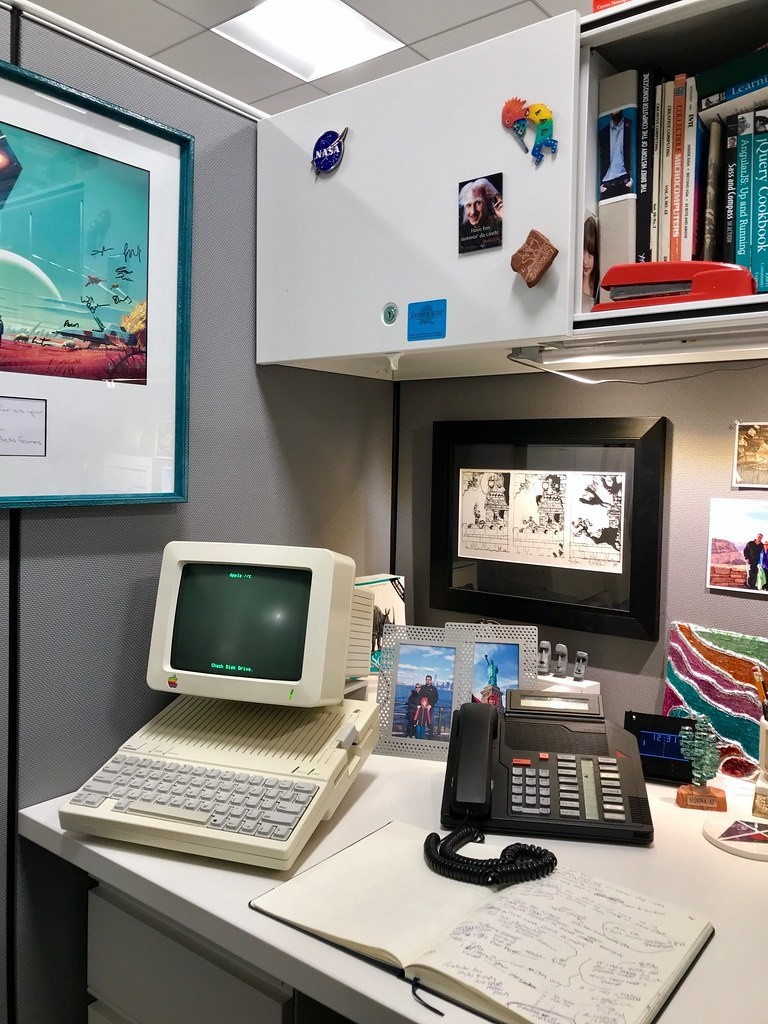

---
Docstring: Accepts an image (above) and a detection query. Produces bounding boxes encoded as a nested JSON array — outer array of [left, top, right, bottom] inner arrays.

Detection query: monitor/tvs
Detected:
[[146, 539, 375, 709]]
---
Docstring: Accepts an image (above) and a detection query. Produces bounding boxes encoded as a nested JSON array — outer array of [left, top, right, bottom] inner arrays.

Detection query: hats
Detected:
[[421, 697, 428, 703], [763, 541, 768, 544], [487, 695, 498, 706]]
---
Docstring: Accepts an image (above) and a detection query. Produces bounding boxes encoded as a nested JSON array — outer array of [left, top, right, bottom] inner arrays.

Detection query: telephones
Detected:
[[439, 700, 656, 848]]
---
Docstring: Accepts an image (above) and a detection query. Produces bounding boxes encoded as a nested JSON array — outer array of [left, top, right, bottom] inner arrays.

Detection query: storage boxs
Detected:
[[354, 572, 406, 672]]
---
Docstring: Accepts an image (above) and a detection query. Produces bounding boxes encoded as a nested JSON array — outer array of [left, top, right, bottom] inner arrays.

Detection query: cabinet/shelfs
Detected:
[[254, 0, 768, 383]]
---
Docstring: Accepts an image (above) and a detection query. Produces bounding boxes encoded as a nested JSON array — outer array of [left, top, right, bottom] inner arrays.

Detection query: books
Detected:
[[598, 45, 768, 304], [248, 821, 715, 1024]]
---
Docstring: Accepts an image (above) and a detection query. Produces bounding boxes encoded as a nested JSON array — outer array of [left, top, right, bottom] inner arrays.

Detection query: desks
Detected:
[[17, 753, 768, 1024]]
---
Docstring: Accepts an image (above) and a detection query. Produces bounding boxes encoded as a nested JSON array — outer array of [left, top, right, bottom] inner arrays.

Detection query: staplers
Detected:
[[589, 261, 757, 314]]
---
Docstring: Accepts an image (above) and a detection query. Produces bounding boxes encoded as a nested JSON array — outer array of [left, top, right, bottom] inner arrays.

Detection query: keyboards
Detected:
[[59, 730, 350, 870]]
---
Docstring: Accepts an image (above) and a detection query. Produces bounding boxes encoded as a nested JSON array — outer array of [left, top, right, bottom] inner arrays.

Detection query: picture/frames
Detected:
[[445, 622, 538, 712], [374, 623, 476, 759], [0, 59, 196, 508], [429, 415, 666, 643]]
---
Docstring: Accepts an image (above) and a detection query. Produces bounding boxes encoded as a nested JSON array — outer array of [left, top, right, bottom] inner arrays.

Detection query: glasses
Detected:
[[416, 687, 420, 688]]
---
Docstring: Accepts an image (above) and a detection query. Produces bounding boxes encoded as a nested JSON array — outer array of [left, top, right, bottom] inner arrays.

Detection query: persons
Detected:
[[459, 178, 503, 253], [582, 216, 598, 298], [743, 534, 768, 591], [485, 655, 498, 687], [406, 675, 438, 739], [598, 110, 633, 201]]
